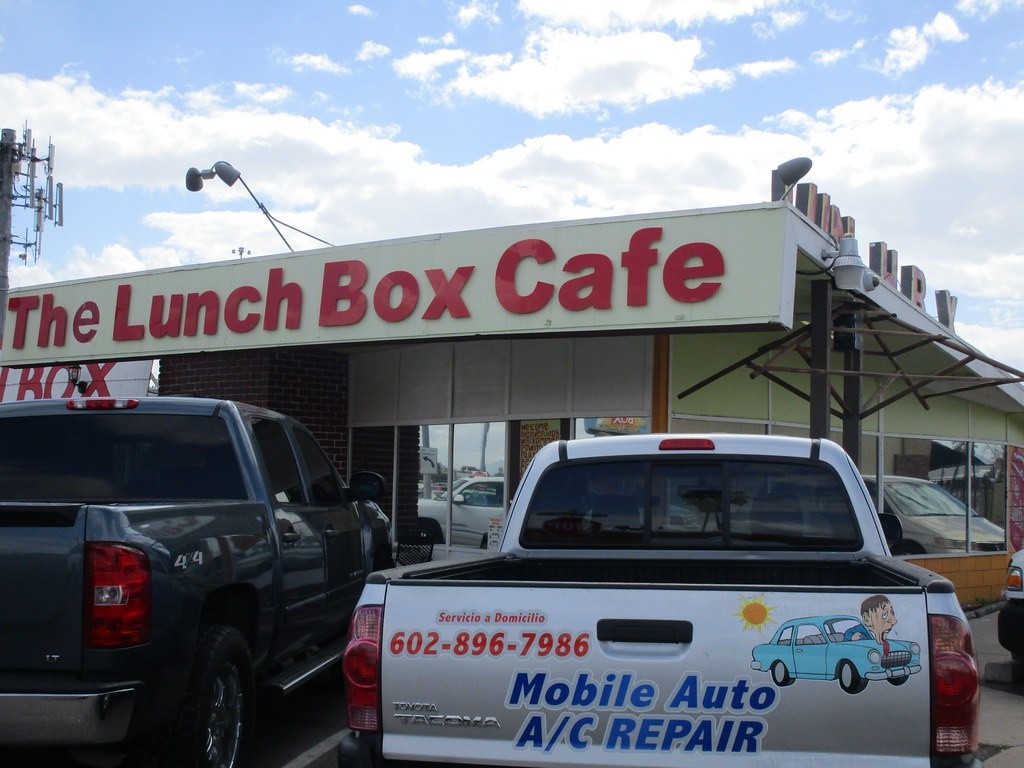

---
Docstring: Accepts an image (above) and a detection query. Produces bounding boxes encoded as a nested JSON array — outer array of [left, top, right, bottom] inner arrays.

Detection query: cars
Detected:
[[997, 548, 1024, 660], [782, 469, 1008, 558], [418, 471, 507, 552]]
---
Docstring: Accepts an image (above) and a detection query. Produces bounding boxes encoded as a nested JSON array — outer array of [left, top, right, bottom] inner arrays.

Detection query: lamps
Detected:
[[822, 233, 865, 290], [185, 161, 295, 252], [777, 157, 813, 201], [68, 365, 87, 394]]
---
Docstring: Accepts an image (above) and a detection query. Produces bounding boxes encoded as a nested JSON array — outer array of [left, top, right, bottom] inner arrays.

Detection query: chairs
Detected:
[[748, 481, 838, 539], [396, 535, 435, 566], [588, 496, 645, 535]]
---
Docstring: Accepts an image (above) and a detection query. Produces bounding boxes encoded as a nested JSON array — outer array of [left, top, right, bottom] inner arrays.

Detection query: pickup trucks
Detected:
[[0, 393, 397, 768], [341, 426, 983, 768]]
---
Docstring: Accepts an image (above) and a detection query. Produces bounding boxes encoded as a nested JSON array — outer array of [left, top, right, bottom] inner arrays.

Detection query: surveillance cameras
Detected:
[[863, 273, 880, 293]]
